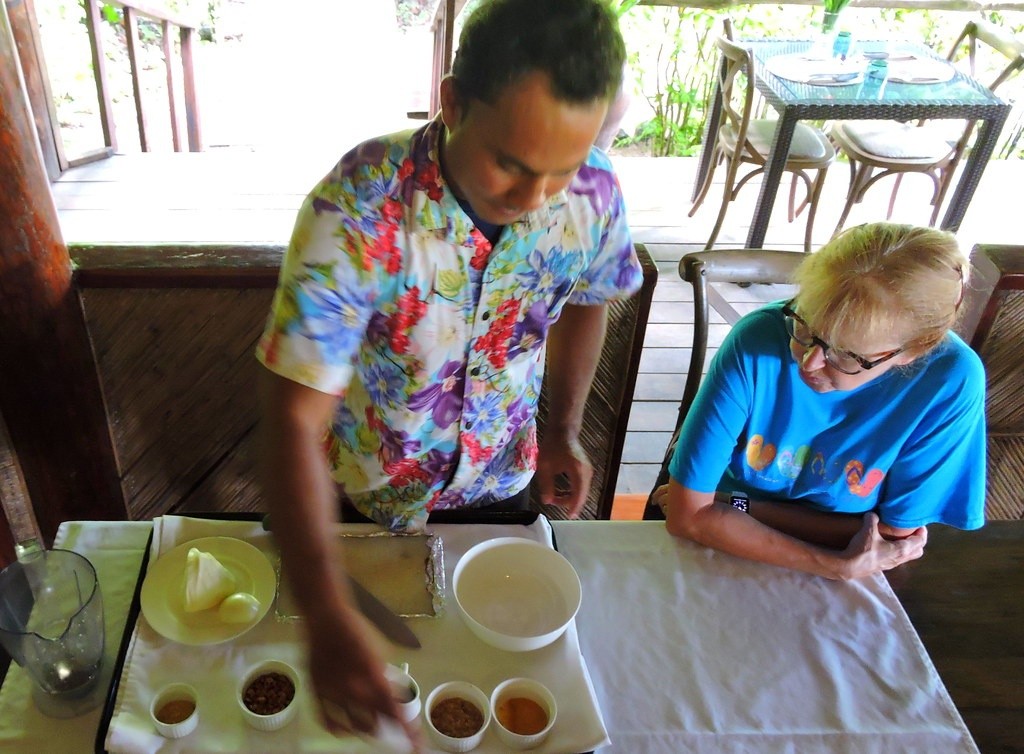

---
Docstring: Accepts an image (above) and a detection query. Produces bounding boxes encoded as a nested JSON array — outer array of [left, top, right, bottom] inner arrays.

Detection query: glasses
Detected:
[[782, 291, 909, 376]]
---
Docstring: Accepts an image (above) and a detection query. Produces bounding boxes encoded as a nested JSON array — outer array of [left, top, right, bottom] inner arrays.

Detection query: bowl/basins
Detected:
[[450, 536, 583, 652]]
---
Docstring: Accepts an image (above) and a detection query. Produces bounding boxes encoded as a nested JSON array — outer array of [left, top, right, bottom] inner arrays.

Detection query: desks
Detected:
[[691, 38, 1013, 287], [0, 518, 1024, 754]]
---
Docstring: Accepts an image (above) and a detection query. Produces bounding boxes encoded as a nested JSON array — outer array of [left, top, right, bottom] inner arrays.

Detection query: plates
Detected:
[[139, 537, 277, 647]]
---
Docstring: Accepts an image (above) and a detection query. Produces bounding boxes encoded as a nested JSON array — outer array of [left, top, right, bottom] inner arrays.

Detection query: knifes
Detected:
[[263, 512, 422, 650]]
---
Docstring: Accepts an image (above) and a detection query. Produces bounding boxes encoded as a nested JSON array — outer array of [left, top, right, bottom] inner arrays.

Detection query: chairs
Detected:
[[688, 38, 836, 252], [810, 21, 1024, 242], [642, 249, 808, 519]]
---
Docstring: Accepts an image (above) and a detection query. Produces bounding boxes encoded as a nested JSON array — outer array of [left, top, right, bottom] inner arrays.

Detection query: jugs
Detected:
[[0, 548, 109, 720]]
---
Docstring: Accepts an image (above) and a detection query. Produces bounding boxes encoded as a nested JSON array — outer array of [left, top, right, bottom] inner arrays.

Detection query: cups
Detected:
[[424, 681, 491, 750], [235, 659, 301, 733], [384, 663, 421, 724], [149, 681, 200, 739], [491, 677, 558, 750]]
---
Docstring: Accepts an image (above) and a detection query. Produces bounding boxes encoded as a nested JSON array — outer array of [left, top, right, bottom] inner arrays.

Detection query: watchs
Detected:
[[729, 491, 750, 516]]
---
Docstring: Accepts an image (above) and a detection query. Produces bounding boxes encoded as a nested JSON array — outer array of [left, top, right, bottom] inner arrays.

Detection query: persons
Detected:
[[255, 0, 643, 754], [644, 221, 987, 582]]
